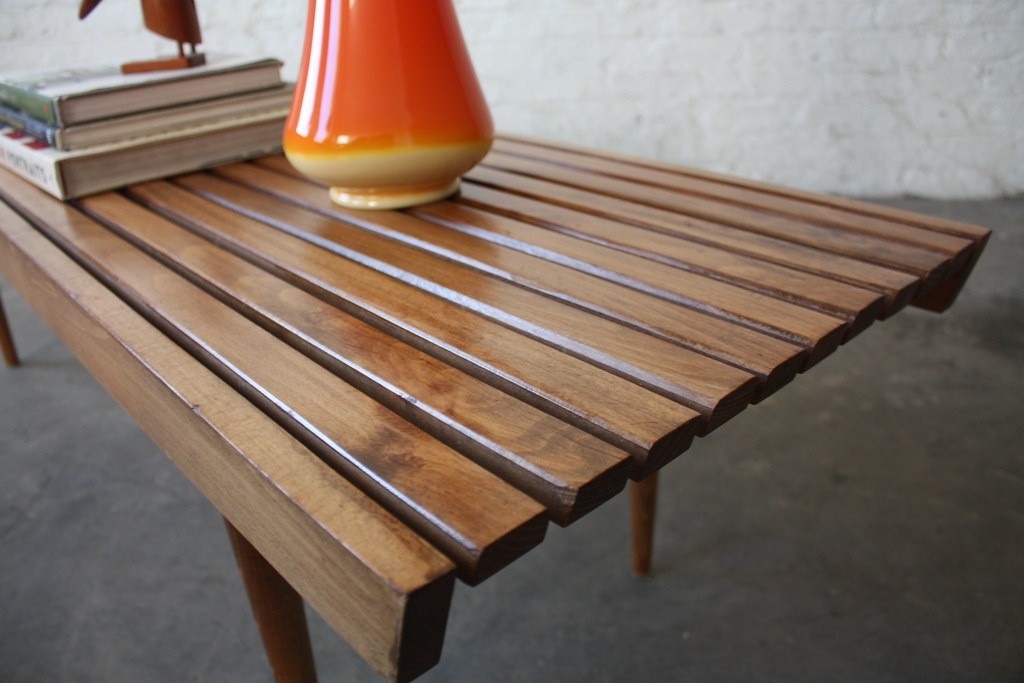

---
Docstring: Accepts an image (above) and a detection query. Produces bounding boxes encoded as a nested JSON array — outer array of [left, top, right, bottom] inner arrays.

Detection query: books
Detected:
[[0, 57, 298, 203]]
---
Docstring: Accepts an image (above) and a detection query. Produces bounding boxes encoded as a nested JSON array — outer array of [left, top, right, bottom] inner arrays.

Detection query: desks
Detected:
[[1, 129, 992, 683]]
